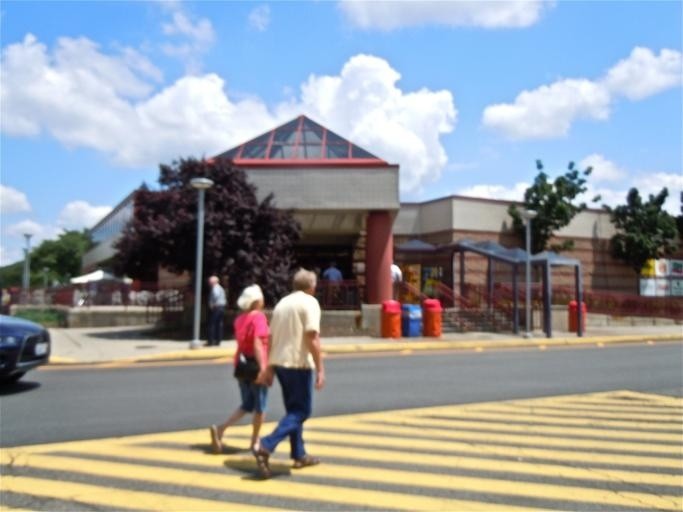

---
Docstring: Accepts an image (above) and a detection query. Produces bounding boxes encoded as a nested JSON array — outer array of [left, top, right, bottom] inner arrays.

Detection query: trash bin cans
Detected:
[[380, 300, 443, 337], [569, 301, 586, 332]]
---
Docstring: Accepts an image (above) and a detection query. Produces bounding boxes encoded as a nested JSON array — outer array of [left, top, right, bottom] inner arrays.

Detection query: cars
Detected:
[[0, 314, 51, 397]]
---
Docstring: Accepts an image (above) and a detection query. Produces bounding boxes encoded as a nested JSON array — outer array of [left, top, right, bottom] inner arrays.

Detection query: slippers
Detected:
[[209, 424, 224, 454]]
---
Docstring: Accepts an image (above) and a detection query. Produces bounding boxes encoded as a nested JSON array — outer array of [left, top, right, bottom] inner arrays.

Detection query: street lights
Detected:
[[22, 233, 32, 289], [520, 209, 537, 338], [189, 177, 214, 349]]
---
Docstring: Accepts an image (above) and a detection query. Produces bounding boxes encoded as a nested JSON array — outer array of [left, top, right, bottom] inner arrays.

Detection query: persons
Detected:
[[390, 260, 403, 301], [325, 260, 343, 305], [200, 276, 227, 348], [208, 268, 326, 478]]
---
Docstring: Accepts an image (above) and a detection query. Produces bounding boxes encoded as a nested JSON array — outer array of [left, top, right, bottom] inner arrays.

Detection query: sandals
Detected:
[[293, 453, 322, 470], [253, 444, 272, 479]]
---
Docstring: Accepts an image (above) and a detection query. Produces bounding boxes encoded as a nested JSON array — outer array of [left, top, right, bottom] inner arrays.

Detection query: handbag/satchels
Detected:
[[234, 353, 259, 380]]
